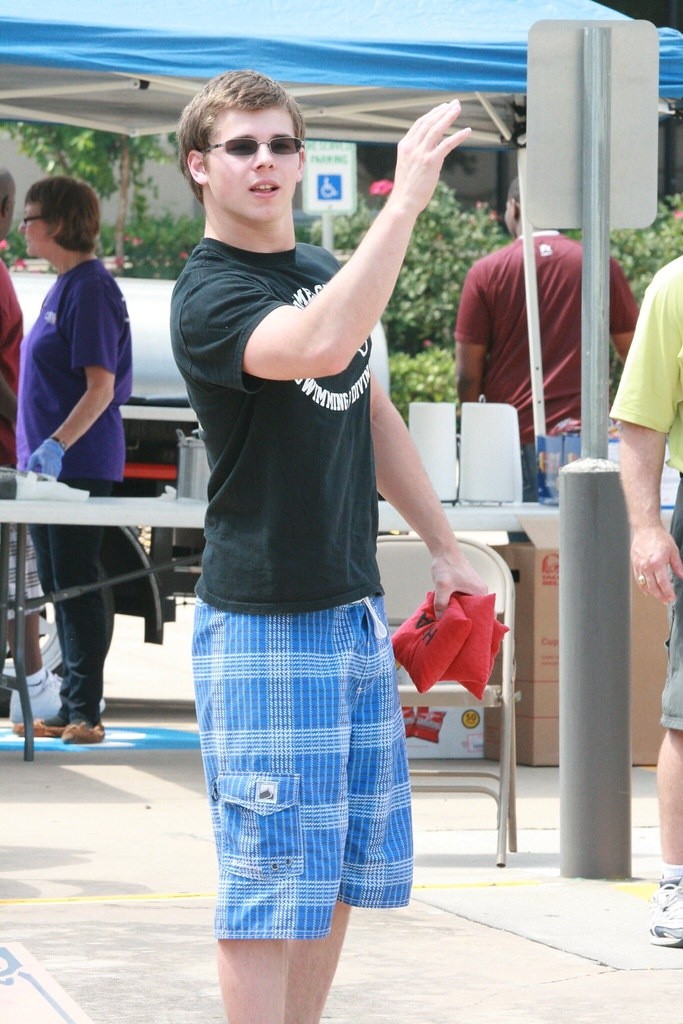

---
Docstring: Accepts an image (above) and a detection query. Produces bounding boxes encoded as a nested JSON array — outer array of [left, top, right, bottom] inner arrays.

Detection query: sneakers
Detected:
[[649, 876, 683, 945], [10, 670, 104, 723]]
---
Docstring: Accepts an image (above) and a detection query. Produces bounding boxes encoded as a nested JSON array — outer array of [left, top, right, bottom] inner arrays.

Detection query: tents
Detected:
[[0, 0, 683, 466]]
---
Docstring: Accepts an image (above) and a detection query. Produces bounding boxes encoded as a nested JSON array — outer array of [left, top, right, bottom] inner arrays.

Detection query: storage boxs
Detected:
[[535, 435, 564, 505], [393, 660, 483, 757], [560, 437, 680, 508], [483, 515, 672, 769]]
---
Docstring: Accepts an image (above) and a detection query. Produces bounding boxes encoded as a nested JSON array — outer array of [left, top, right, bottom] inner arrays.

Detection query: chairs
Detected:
[[370, 535, 525, 869]]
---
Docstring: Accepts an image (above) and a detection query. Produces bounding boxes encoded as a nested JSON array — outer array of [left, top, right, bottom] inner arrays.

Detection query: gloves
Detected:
[[25, 438, 63, 481]]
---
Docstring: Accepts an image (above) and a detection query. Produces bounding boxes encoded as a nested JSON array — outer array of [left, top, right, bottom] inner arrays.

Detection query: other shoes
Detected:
[[13, 719, 64, 737], [61, 723, 104, 744]]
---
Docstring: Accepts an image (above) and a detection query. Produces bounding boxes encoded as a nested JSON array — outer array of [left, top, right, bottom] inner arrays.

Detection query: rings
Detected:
[[638, 575, 646, 585]]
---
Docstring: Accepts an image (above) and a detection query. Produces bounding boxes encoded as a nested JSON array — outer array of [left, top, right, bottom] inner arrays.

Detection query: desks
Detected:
[[0, 495, 674, 761]]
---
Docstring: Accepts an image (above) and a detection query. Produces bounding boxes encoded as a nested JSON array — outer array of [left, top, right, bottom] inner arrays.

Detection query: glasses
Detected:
[[201, 137, 303, 155], [23, 215, 45, 223]]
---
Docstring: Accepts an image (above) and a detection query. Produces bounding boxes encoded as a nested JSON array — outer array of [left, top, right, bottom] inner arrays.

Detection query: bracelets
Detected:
[[51, 436, 66, 452]]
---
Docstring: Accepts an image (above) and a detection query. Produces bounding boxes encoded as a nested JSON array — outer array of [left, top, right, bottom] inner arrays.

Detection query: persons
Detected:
[[454, 177, 639, 545], [15, 177, 133, 744], [0, 168, 105, 724], [608, 255, 683, 949], [169, 69, 489, 1023]]
